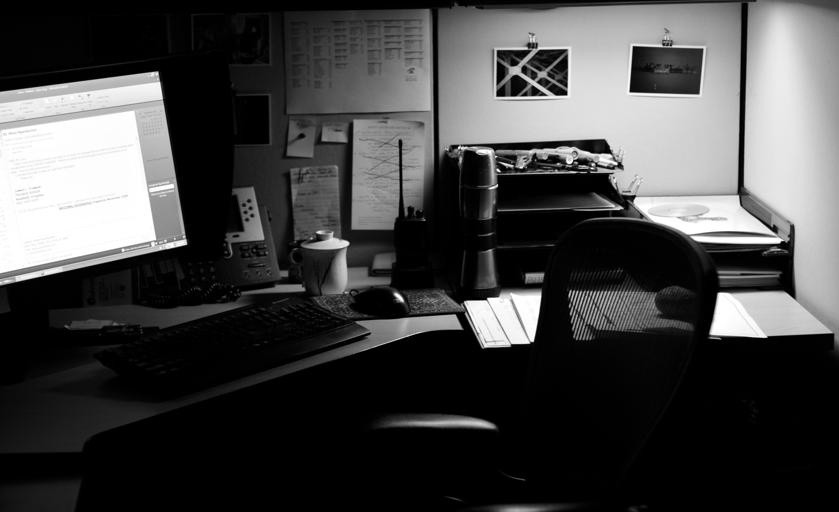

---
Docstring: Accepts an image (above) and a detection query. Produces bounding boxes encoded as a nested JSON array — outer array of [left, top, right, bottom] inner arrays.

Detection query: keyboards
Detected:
[[104, 292, 372, 402]]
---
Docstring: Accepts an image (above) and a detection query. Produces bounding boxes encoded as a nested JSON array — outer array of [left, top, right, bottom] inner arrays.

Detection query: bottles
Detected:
[[460, 147, 501, 299]]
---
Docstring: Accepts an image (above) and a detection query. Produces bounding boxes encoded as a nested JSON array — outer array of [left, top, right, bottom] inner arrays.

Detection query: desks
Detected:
[[2, 264, 835, 511]]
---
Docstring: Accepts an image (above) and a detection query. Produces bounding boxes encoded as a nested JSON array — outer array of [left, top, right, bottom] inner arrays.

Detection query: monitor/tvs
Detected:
[[0, 55, 193, 385]]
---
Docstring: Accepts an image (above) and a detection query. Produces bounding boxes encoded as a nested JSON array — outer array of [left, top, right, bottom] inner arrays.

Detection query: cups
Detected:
[[289, 230, 350, 293]]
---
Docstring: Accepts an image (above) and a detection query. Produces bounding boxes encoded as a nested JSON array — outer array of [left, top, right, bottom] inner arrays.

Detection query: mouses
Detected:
[[348, 285, 411, 318]]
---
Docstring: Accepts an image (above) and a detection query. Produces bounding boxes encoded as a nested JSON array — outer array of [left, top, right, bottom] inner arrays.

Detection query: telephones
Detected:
[[145, 184, 279, 303]]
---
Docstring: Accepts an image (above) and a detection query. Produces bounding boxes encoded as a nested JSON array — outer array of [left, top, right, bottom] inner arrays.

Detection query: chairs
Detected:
[[365, 218, 718, 511]]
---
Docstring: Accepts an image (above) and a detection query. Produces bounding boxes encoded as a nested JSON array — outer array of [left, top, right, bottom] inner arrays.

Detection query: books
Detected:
[[461, 291, 768, 350]]
